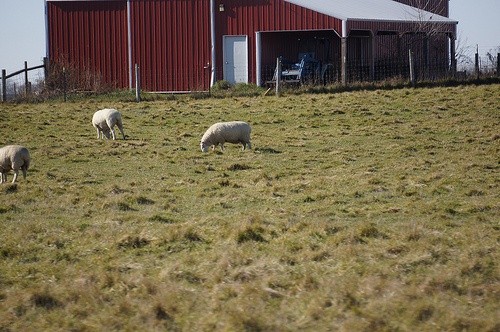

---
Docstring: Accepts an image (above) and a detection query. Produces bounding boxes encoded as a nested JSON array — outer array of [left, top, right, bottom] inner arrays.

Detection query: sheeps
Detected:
[[92, 109, 124, 141], [0, 145, 30, 184], [199, 120, 252, 152]]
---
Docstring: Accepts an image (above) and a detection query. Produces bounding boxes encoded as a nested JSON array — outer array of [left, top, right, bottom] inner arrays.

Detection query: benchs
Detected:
[[263, 54, 307, 97]]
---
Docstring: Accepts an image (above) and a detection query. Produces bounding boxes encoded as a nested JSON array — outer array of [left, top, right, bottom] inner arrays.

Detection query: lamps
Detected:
[[219, 3, 225, 14]]
